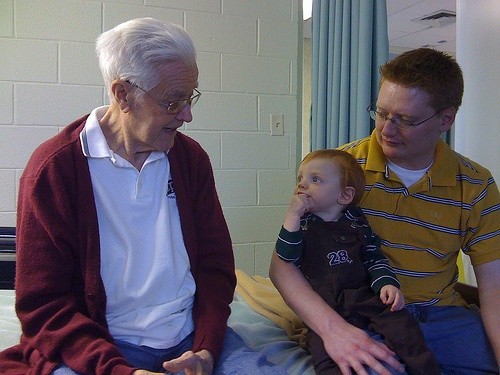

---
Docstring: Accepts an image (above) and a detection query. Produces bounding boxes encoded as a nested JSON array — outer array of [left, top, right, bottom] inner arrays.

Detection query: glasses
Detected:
[[364, 100, 448, 131], [125, 78, 201, 113]]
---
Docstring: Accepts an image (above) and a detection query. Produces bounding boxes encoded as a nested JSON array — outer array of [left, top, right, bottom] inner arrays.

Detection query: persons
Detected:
[[0, 17, 289, 375], [269, 48, 500, 375], [275, 149, 444, 375]]
